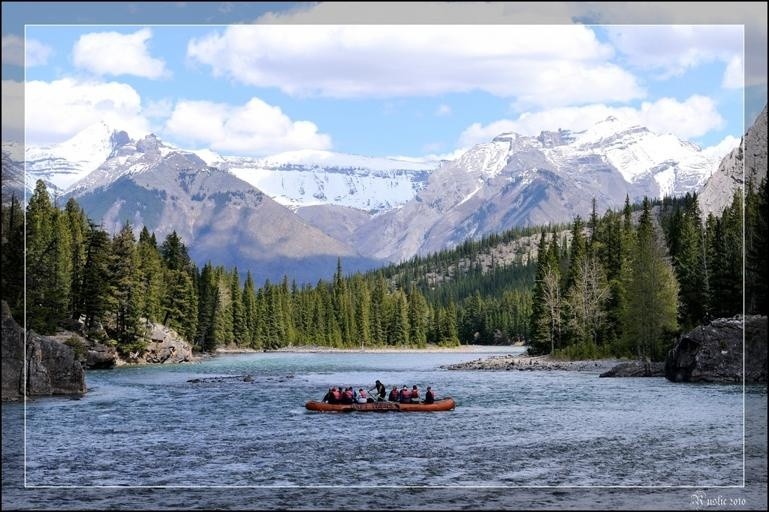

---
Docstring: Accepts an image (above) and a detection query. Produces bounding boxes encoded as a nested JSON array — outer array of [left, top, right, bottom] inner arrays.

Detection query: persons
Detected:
[[322, 380, 434, 404]]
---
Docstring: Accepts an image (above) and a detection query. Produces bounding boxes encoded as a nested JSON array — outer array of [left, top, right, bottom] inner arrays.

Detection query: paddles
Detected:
[[376, 395, 400, 409]]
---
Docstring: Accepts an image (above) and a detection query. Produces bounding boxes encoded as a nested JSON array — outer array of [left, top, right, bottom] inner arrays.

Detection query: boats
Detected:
[[304, 395, 455, 413]]
